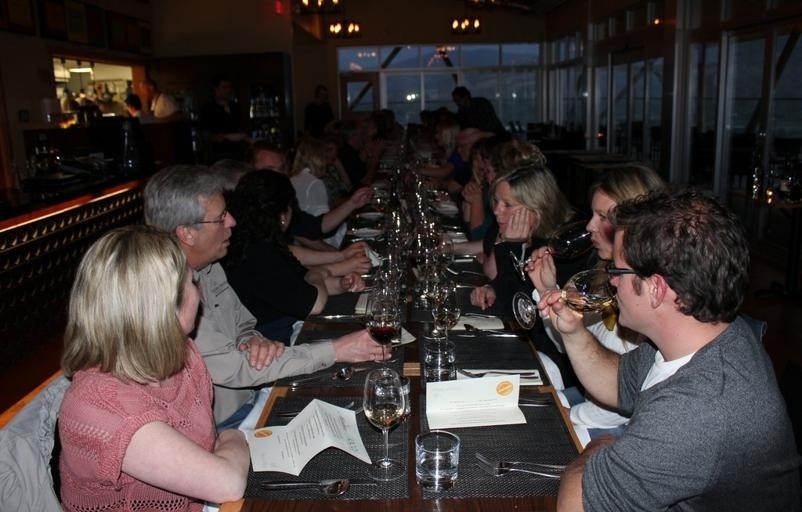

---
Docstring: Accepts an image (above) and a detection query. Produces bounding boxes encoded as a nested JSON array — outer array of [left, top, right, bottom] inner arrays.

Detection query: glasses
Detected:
[[198, 209, 228, 224], [606, 262, 637, 277]]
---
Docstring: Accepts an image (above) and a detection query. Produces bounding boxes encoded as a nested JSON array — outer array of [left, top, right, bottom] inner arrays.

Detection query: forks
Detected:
[[475, 447, 567, 473], [474, 461, 562, 481], [273, 407, 366, 420], [458, 365, 538, 378], [271, 400, 355, 410]]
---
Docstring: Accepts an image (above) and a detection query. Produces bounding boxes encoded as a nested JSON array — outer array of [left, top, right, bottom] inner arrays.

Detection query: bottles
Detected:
[[244, 94, 283, 151], [28, 137, 66, 171]]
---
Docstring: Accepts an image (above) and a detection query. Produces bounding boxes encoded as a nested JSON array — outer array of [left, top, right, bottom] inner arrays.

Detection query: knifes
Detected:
[[262, 477, 379, 492]]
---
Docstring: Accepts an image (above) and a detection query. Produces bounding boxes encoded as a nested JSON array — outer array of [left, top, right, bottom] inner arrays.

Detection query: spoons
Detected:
[[284, 367, 357, 389], [265, 478, 351, 496]]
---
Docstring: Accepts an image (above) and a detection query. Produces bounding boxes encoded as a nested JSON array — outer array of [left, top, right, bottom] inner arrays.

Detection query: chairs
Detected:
[[0, 364, 72, 511]]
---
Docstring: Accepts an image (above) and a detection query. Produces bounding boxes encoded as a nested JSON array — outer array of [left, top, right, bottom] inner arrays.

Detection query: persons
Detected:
[[138, 164, 393, 433], [537, 179, 801, 512], [521, 162, 672, 445], [421, 85, 591, 352], [51, 221, 252, 512], [103, 80, 178, 118], [210, 80, 394, 346]]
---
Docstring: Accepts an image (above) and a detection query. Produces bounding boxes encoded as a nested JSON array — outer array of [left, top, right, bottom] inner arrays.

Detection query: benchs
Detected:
[[0, 178, 146, 341]]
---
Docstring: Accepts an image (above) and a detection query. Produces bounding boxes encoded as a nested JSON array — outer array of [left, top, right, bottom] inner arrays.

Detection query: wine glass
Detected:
[[508, 218, 601, 283], [346, 152, 520, 341], [511, 269, 623, 331], [364, 372, 409, 480], [361, 301, 403, 370]]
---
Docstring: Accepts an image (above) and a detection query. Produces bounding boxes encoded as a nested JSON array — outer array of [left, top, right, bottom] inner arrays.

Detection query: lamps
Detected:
[[59, 58, 101, 114]]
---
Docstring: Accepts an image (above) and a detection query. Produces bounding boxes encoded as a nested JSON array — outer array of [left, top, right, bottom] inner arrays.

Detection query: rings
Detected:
[[369, 354, 375, 361]]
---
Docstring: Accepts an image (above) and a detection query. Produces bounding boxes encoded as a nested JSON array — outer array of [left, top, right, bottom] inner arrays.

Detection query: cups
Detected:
[[419, 337, 460, 383], [416, 428, 461, 491]]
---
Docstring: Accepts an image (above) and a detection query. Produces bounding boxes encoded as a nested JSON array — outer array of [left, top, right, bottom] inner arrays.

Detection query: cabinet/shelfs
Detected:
[[170, 53, 293, 158]]
